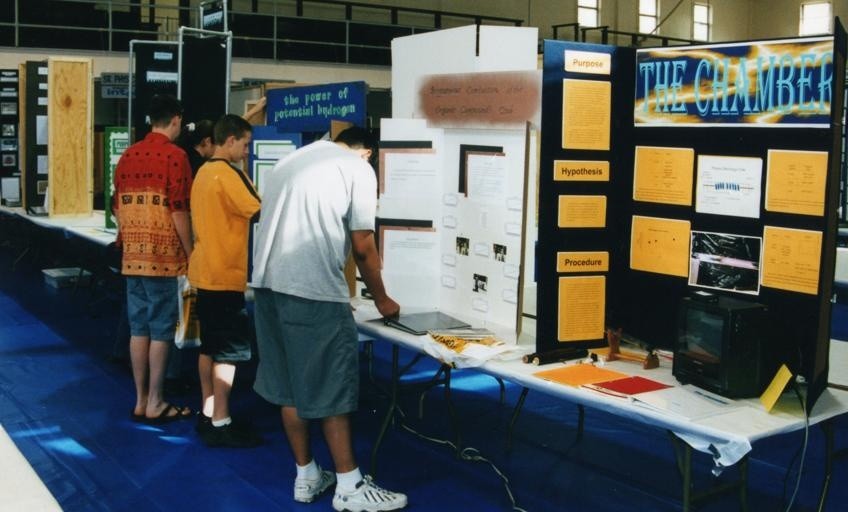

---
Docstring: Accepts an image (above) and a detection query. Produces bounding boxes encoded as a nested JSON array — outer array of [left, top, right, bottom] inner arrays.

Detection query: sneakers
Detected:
[[332, 472, 408, 512], [294, 463, 336, 503], [195, 415, 212, 432], [204, 424, 263, 448]]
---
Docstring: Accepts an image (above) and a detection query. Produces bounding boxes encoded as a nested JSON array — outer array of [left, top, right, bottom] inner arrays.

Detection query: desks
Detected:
[[70, 204, 396, 421], [342, 305, 820, 512], [3, 206, 70, 289]]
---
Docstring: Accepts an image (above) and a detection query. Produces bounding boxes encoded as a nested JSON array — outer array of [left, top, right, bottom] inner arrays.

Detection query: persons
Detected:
[[190, 113, 261, 447], [113, 95, 195, 426], [180, 119, 215, 179], [254, 128, 408, 512]]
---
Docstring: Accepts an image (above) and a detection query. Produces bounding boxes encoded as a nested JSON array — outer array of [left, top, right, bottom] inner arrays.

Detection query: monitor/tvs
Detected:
[[670, 290, 779, 399]]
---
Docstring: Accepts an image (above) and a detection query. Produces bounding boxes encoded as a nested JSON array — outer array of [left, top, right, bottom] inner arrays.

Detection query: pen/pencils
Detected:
[[521, 347, 590, 367]]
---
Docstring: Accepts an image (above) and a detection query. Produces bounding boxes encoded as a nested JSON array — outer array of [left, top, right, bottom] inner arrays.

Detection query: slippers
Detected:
[[144, 402, 193, 424], [129, 406, 144, 422]]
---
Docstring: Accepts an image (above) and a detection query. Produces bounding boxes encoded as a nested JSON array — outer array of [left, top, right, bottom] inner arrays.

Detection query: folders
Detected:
[[628, 383, 740, 421], [383, 311, 472, 335]]
